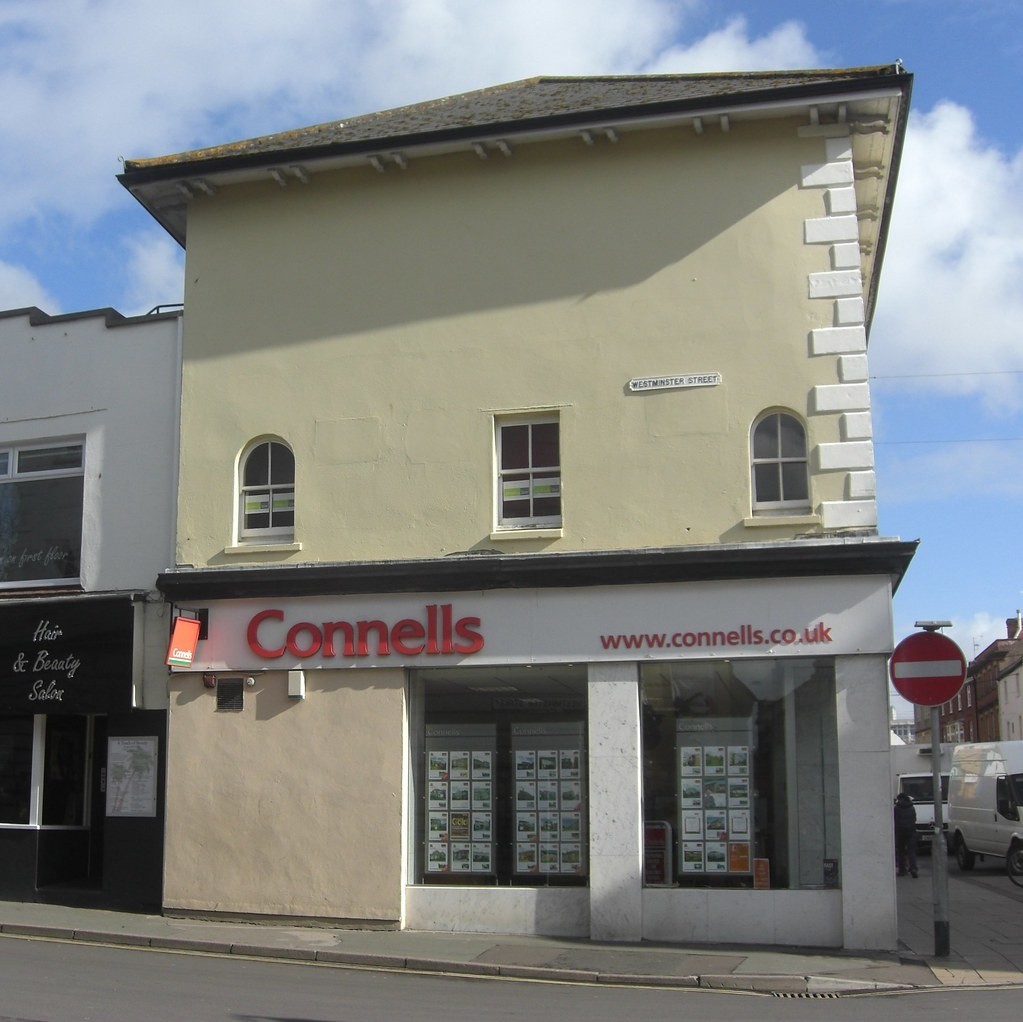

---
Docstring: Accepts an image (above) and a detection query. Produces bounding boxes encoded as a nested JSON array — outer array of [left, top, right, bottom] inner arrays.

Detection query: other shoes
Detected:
[[896, 869, 906, 875], [911, 870, 918, 876]]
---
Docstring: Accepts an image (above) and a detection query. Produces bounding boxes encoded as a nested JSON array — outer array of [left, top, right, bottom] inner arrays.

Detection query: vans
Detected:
[[945, 739, 1023, 886], [895, 772, 950, 856]]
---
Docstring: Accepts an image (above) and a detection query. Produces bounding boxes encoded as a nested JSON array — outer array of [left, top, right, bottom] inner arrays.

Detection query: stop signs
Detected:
[[888, 632, 968, 707]]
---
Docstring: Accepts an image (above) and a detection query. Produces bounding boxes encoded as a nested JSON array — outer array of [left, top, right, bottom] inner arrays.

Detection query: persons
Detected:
[[893, 792, 920, 879]]
